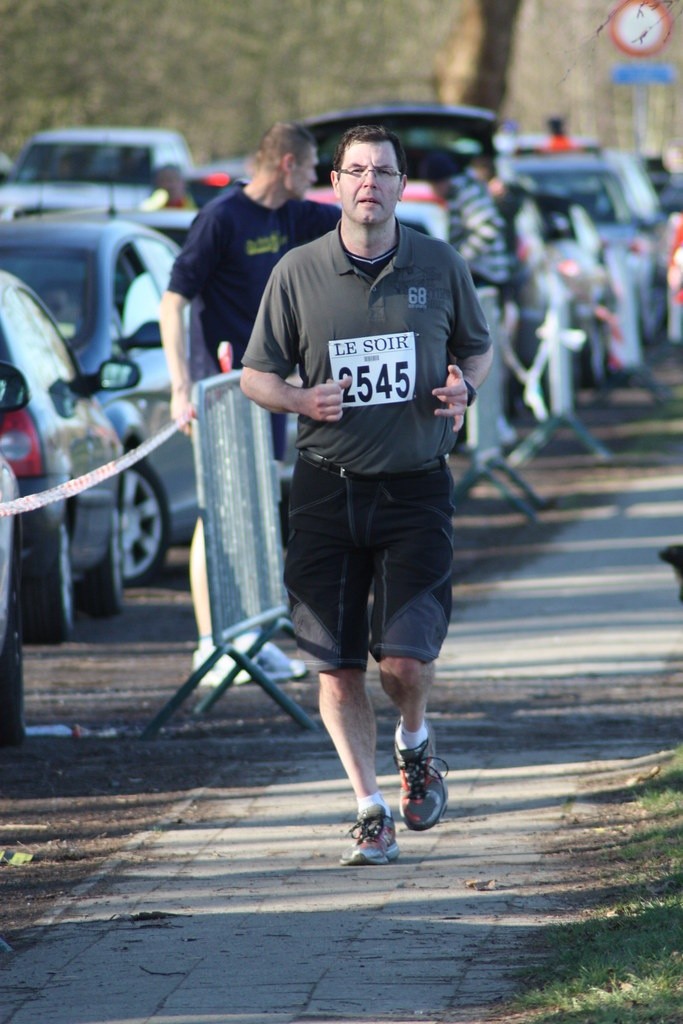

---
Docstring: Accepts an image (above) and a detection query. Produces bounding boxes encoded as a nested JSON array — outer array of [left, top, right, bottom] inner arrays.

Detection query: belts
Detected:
[[298, 448, 451, 479]]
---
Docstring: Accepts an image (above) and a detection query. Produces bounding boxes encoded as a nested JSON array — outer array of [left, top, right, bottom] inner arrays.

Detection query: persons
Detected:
[[59, 153, 73, 178], [535, 117, 580, 153], [488, 177, 524, 222], [142, 163, 194, 211], [238, 125, 493, 865], [158, 120, 341, 686], [445, 151, 508, 286]]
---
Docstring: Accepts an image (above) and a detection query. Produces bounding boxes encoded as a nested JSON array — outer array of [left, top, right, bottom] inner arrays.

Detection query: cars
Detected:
[[0, 103, 679, 753]]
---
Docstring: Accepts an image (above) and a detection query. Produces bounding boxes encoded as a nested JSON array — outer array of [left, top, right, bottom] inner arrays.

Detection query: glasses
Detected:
[[339, 166, 402, 181]]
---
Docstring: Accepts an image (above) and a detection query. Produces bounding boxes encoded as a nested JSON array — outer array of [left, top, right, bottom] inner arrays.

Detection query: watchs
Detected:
[[464, 380, 477, 407]]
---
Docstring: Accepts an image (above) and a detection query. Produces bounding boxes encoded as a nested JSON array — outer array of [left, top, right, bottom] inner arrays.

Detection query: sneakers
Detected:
[[192, 646, 253, 685], [339, 804, 399, 865], [240, 642, 307, 682], [393, 718, 449, 831]]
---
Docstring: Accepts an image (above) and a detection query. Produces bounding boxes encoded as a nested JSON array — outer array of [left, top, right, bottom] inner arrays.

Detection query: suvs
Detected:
[[0, 125, 197, 226]]
